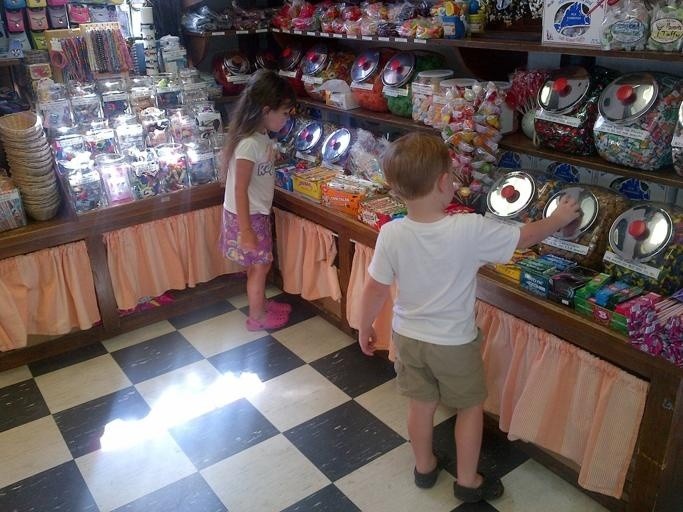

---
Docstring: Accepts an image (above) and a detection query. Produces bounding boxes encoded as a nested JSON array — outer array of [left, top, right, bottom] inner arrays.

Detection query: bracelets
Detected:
[[237, 229, 252, 235]]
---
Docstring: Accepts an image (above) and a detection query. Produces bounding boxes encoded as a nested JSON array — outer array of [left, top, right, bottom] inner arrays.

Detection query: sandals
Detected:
[[453, 471, 504, 502], [245, 299, 293, 331], [414, 446, 450, 489]]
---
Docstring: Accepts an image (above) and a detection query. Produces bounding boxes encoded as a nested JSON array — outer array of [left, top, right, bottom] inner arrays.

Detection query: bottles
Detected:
[[39, 67, 228, 215], [594, 69, 683, 179], [200, 44, 357, 102], [349, 47, 401, 113], [380, 49, 448, 121], [485, 169, 568, 250], [411, 68, 513, 136], [536, 179, 631, 271], [604, 198, 683, 295], [534, 63, 620, 161], [484, 0, 683, 49], [269, 115, 358, 169]]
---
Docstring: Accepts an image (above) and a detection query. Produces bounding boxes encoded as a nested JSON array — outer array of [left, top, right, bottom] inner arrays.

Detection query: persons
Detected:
[[220, 71, 296, 330], [359, 132, 580, 504]]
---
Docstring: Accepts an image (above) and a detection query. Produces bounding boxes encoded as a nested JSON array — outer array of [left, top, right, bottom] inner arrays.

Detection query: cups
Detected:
[[441, 13, 458, 39], [468, 13, 485, 34]]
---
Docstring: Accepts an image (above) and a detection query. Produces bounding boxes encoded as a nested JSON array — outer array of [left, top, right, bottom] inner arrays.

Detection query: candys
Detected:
[[39, 0, 683, 367]]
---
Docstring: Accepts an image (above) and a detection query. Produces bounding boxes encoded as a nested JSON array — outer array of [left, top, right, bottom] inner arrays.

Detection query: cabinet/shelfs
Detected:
[[2, 2, 266, 352], [176, 2, 683, 509]]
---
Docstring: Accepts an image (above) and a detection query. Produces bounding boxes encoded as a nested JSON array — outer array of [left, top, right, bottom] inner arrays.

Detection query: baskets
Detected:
[[0, 110, 62, 221]]
